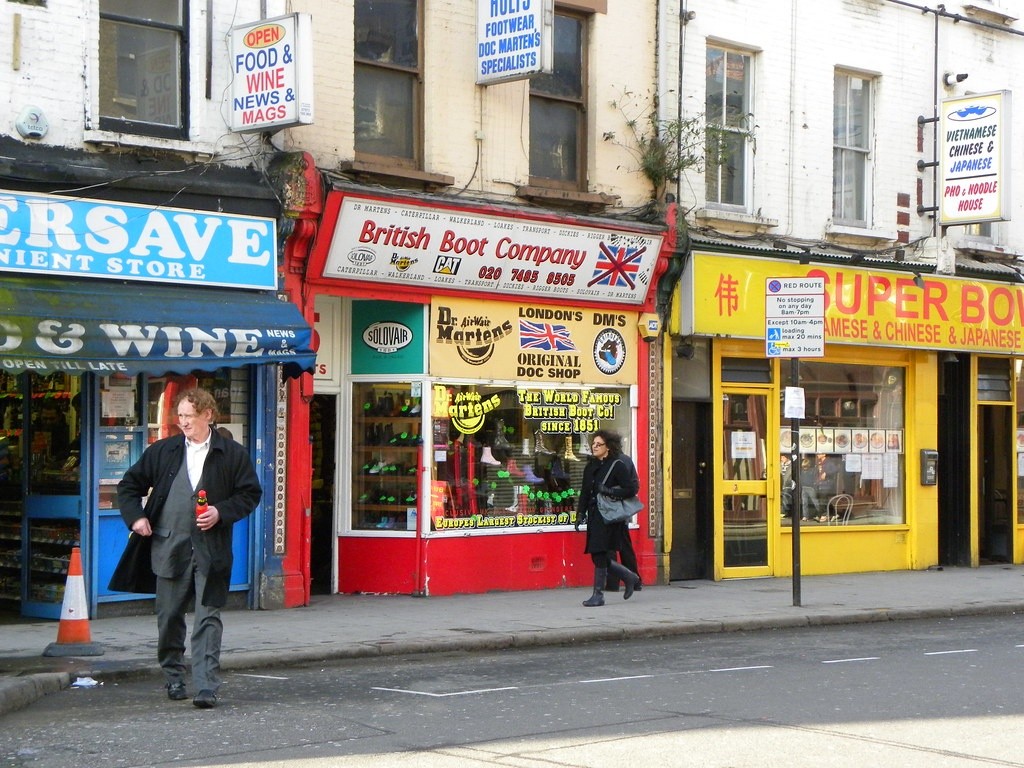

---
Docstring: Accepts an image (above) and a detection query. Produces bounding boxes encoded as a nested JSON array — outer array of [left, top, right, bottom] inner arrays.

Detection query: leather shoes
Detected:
[[167, 681, 187, 700], [193, 689, 217, 709]]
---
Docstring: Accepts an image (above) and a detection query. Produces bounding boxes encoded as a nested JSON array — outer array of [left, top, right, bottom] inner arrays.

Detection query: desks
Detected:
[[826, 495, 878, 518]]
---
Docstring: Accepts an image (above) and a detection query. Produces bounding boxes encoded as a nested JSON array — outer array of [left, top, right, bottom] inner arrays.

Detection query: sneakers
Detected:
[[358, 389, 424, 528]]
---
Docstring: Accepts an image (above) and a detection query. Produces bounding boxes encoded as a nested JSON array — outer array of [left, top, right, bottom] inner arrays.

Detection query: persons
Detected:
[[32, 392, 81, 466], [780, 454, 840, 523], [117, 388, 263, 710], [575, 429, 643, 607]]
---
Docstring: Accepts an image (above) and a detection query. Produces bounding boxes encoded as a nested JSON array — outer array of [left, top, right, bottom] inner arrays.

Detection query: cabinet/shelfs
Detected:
[[0, 496, 81, 610], [351, 382, 422, 529]]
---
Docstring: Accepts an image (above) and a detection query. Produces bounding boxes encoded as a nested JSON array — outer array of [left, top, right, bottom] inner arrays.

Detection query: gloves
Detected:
[[574, 513, 585, 532], [597, 482, 614, 496]]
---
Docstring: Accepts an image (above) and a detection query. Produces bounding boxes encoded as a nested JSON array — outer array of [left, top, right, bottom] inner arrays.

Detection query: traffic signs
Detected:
[[765, 277, 825, 358]]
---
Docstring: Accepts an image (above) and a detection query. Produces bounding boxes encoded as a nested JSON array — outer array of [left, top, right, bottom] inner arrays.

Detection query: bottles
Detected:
[[196, 490, 208, 518]]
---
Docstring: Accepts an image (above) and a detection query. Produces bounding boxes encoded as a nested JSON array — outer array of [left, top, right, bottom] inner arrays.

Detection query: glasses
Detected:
[[592, 441, 606, 447]]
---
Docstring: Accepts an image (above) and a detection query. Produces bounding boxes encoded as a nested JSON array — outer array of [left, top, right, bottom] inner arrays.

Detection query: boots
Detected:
[[479, 419, 593, 516], [607, 559, 640, 600], [583, 567, 606, 606]]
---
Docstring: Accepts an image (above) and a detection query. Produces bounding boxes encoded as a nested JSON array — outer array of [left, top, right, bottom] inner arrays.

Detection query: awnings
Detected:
[[0, 271, 319, 380]]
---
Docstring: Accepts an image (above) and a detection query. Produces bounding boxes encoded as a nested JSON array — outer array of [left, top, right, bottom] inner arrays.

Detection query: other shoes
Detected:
[[783, 512, 791, 518], [802, 516, 808, 521], [633, 583, 643, 591], [814, 516, 820, 522], [831, 515, 840, 522], [819, 516, 828, 522], [605, 586, 619, 592]]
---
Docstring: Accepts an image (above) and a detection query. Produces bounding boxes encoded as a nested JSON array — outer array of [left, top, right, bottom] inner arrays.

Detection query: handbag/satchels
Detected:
[[597, 458, 644, 524]]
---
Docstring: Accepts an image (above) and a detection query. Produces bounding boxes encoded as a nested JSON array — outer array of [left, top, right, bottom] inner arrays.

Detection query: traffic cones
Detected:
[[42, 546, 105, 657]]
